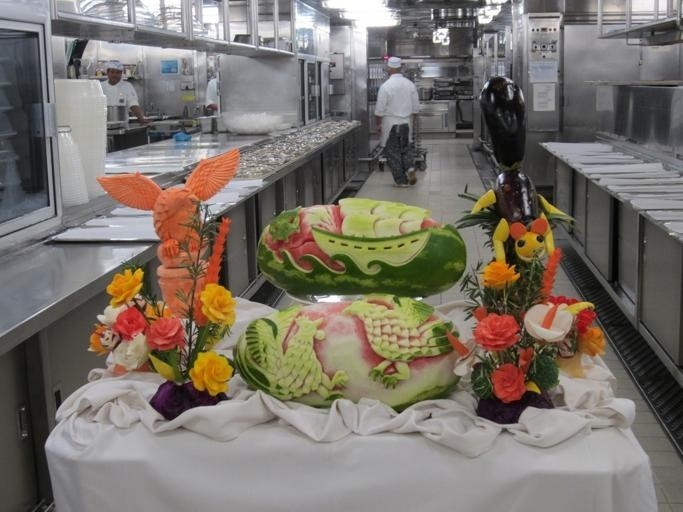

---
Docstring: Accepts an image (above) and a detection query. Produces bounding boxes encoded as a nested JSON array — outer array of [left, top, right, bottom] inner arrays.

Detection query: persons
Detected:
[[205, 78, 218, 115], [100, 60, 149, 123], [374, 56, 420, 187]]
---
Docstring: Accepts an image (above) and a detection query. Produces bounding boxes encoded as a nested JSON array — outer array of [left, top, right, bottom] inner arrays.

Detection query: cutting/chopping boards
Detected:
[[584, 81, 682, 88]]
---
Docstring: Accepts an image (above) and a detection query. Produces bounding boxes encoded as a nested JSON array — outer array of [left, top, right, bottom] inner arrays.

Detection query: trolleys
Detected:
[[376, 104, 428, 173]]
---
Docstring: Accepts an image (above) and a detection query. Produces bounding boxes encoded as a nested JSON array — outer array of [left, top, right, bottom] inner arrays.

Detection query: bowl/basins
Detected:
[[52, 80, 107, 208]]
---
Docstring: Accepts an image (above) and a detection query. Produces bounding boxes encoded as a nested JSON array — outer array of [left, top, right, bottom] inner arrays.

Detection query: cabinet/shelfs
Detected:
[[542, 134, 683, 380], [0, 118, 366, 510], [0, 0, 62, 241], [367, 100, 456, 133]]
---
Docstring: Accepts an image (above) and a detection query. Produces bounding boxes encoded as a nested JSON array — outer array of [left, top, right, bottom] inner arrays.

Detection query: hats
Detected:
[[106, 59, 123, 70], [387, 56, 402, 69]]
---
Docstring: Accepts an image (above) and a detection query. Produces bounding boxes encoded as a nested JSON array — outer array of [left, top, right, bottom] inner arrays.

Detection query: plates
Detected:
[[85, 180, 263, 226], [44, 227, 161, 242], [541, 141, 683, 242]]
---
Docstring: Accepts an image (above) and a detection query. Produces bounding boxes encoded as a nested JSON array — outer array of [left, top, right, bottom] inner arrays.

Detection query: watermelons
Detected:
[[232, 293, 469, 414], [256, 198, 466, 298]]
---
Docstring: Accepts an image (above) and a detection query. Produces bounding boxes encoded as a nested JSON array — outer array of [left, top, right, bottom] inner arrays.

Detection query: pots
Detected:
[[107, 105, 129, 130]]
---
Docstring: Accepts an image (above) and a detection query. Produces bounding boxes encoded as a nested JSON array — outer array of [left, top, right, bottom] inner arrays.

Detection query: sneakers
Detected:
[[394, 168, 418, 187]]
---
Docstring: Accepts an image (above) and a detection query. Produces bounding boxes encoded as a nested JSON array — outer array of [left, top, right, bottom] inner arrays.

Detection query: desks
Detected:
[[44, 347, 647, 511]]
